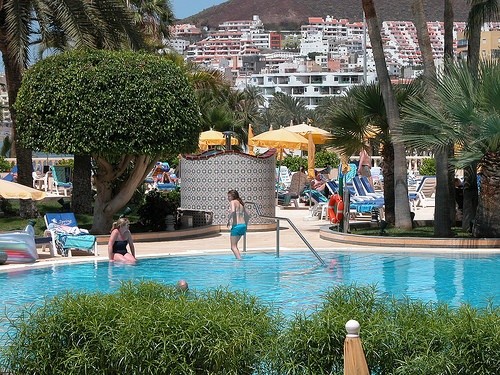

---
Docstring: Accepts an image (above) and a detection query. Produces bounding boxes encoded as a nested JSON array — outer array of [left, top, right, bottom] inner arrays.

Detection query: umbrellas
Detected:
[[343, 319, 370, 375], [0, 179, 45, 201], [199, 122, 390, 207]]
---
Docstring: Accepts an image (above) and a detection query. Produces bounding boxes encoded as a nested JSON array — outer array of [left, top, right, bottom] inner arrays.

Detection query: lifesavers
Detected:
[[328, 194, 344, 223]]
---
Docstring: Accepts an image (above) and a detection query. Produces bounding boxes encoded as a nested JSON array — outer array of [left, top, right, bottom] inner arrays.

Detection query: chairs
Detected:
[[303, 176, 436, 220], [42, 213, 98, 257]]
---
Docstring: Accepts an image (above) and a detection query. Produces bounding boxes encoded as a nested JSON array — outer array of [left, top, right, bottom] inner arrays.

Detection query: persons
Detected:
[[226, 190, 247, 258], [108, 218, 137, 261], [358, 149, 372, 182], [177, 280, 188, 288]]
